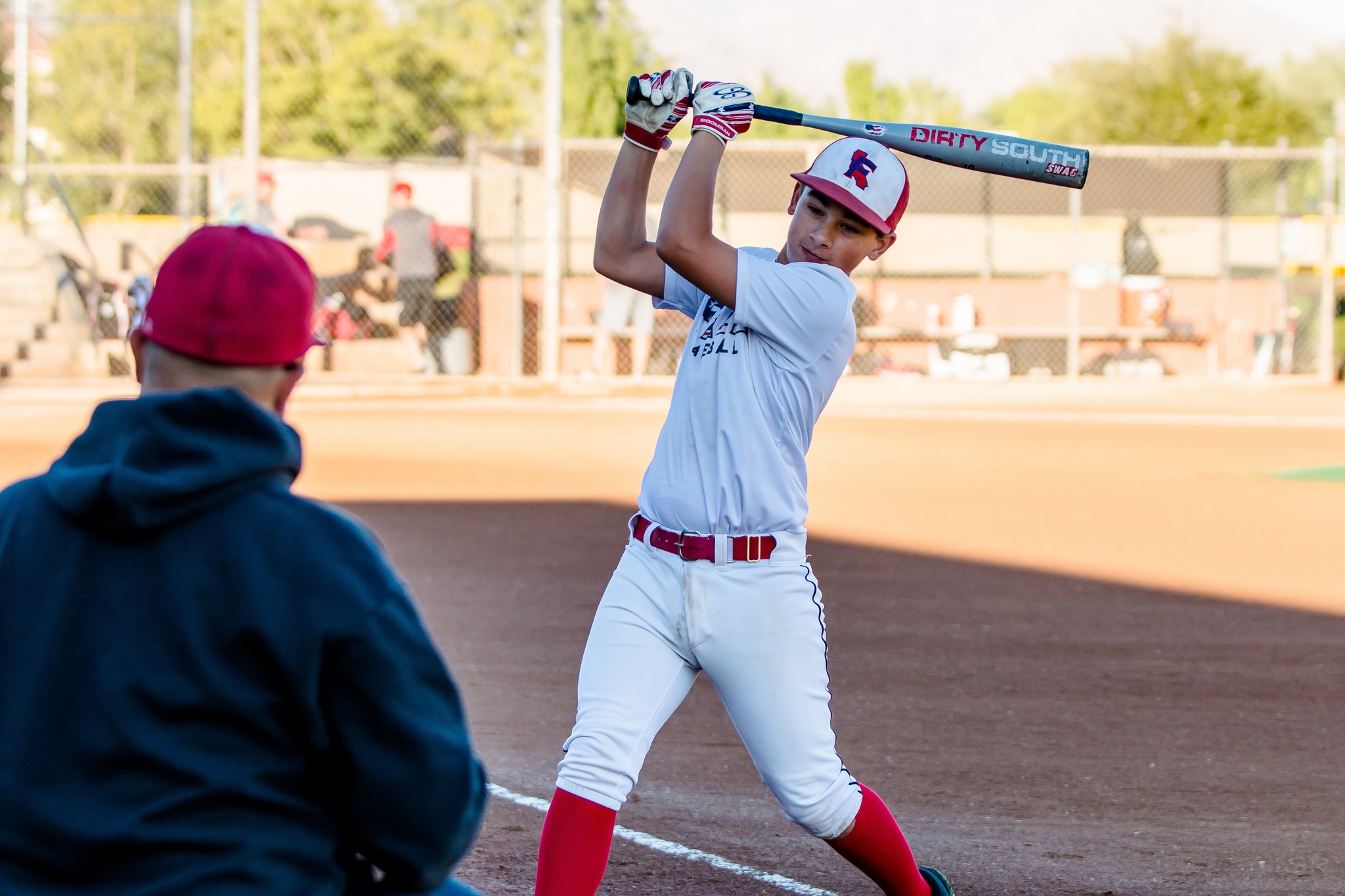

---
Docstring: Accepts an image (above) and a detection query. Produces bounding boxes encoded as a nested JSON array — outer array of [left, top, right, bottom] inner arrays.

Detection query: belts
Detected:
[[632, 515, 777, 563]]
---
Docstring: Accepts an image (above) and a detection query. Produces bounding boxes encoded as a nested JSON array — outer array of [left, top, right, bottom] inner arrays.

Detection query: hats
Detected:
[[390, 180, 411, 197], [138, 221, 331, 369], [790, 137, 910, 235]]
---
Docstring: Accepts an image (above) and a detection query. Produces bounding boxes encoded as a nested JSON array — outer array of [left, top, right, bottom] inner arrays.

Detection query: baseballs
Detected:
[[626, 76, 1089, 190]]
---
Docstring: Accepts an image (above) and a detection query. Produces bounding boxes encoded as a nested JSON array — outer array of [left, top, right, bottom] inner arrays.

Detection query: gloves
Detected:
[[692, 80, 755, 146], [623, 67, 694, 152]]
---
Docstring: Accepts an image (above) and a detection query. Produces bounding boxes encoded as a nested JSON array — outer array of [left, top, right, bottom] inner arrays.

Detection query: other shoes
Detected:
[[916, 864, 955, 896]]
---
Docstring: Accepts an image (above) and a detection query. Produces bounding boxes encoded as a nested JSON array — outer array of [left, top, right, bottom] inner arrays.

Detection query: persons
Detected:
[[534, 69, 954, 896], [1, 223, 490, 896], [320, 185, 462, 345], [111, 246, 148, 344]]
